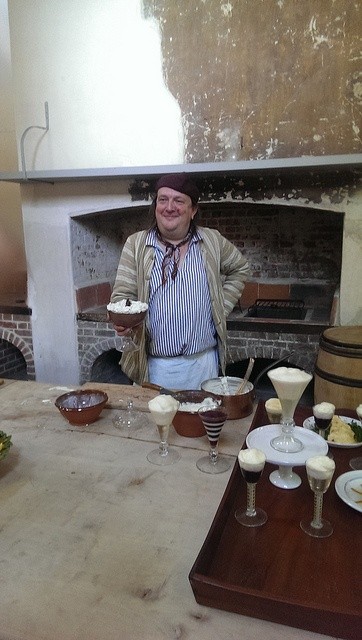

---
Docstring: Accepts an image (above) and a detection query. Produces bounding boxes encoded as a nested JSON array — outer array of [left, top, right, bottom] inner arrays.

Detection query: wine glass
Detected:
[[147, 402, 184, 466], [267, 368, 313, 453], [313, 403, 334, 440], [301, 456, 335, 537], [265, 401, 283, 424], [235, 448, 269, 526], [197, 407, 232, 473]]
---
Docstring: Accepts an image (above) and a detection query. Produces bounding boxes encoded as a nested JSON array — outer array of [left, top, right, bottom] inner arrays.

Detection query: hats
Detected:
[[156, 173, 201, 202]]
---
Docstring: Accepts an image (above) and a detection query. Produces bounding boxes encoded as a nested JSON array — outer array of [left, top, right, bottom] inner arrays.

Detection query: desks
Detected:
[[0, 379, 362, 640]]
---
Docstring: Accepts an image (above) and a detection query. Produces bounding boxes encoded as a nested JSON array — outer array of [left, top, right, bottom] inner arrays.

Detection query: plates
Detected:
[[303, 415, 362, 448], [335, 469, 361, 512]]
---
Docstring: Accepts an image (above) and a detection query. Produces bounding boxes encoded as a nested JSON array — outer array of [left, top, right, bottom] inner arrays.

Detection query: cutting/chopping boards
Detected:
[[81, 381, 179, 411]]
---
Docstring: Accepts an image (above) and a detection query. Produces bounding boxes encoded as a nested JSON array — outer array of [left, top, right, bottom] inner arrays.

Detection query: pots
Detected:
[[201, 354, 294, 420]]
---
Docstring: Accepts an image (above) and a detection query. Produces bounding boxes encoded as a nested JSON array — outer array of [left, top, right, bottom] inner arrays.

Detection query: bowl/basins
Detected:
[[55, 389, 106, 425], [107, 300, 147, 326], [169, 389, 222, 437]]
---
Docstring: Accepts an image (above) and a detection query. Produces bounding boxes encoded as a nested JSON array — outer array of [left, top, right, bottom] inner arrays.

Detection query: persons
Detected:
[[107, 173, 251, 394]]
